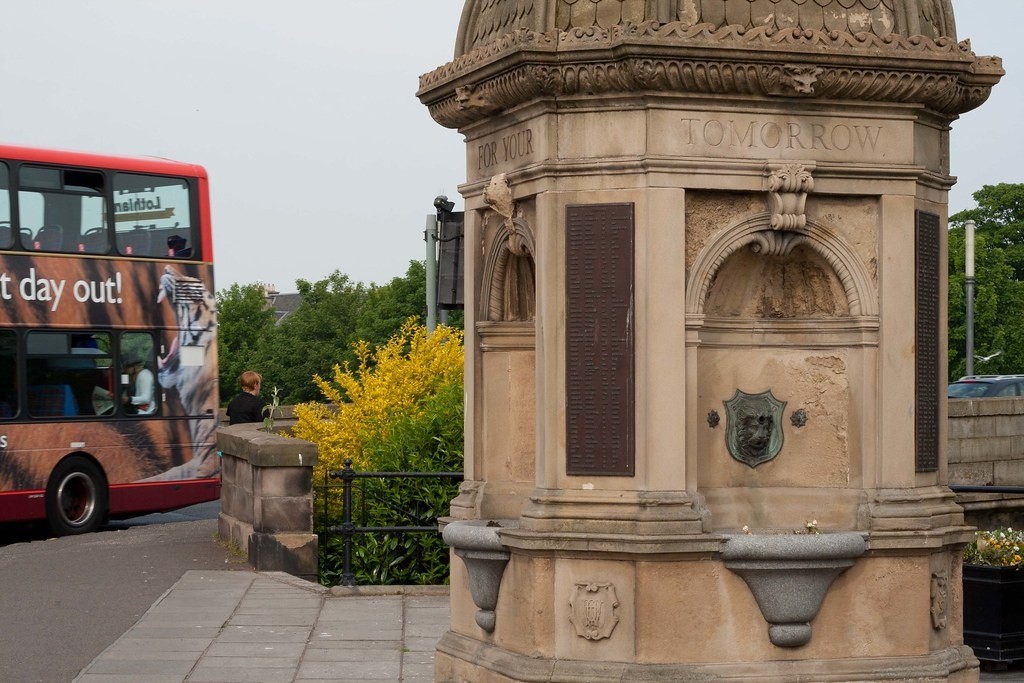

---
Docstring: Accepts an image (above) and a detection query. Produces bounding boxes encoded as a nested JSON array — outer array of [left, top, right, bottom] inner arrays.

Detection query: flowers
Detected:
[[963, 526, 1024, 571]]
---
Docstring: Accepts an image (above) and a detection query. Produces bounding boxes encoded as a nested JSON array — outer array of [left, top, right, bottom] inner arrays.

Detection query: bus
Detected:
[[0, 142, 221, 535]]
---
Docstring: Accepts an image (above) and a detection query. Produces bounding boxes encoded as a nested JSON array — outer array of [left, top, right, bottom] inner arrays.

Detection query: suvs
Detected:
[[947, 375, 1024, 398]]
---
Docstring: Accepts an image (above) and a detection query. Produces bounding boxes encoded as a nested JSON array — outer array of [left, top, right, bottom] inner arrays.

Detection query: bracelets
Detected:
[[128, 396, 132, 402]]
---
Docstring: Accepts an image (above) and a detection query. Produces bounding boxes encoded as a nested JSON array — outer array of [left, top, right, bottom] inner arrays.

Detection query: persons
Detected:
[[225, 370, 269, 426], [120, 353, 157, 415]]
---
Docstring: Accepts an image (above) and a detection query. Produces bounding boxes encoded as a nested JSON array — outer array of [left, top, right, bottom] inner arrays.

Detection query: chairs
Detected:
[[0, 221, 151, 256]]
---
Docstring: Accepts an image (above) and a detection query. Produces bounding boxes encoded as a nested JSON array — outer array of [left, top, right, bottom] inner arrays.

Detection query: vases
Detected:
[[963, 564, 1024, 661]]
